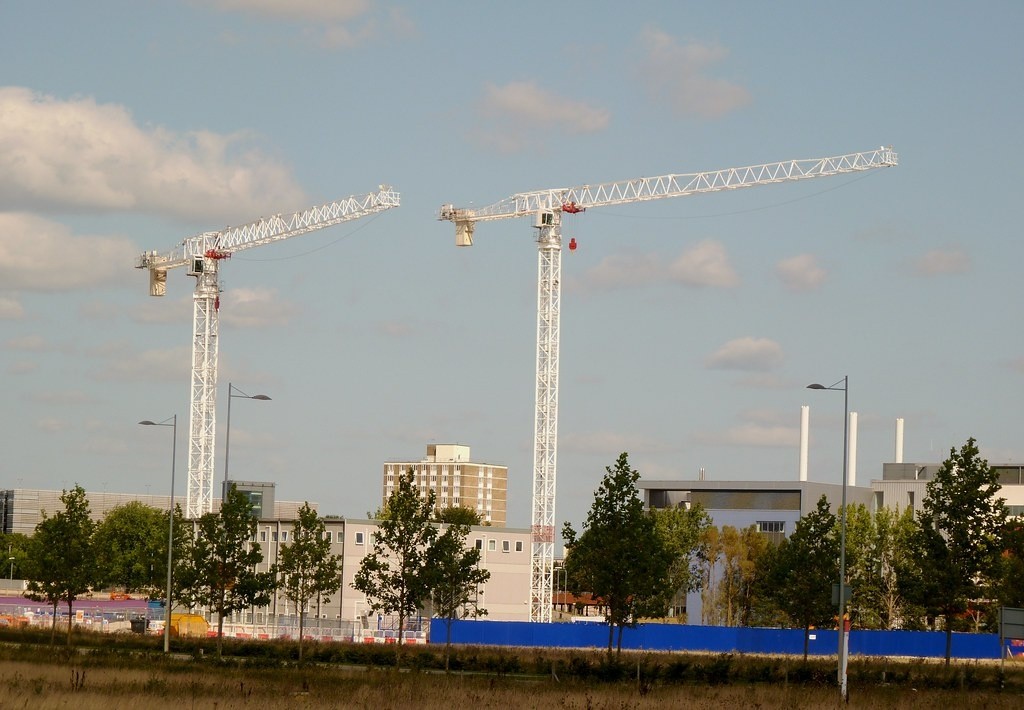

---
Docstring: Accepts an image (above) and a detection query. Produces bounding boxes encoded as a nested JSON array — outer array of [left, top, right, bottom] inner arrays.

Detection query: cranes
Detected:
[[436, 146, 903, 635], [124, 183, 408, 616]]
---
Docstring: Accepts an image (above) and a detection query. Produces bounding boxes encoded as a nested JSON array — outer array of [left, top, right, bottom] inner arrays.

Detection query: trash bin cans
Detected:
[[162, 613, 210, 639], [130, 617, 150, 633]]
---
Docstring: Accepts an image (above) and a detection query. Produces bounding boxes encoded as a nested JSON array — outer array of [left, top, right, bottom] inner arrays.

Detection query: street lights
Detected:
[[215, 381, 270, 659], [134, 416, 180, 657], [804, 375, 850, 685]]
